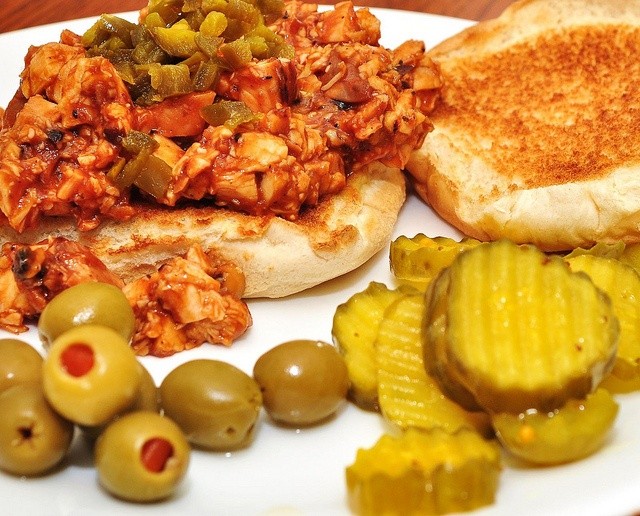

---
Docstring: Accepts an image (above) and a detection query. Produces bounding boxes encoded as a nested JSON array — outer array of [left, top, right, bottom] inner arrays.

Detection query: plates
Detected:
[[0, 5, 640, 516]]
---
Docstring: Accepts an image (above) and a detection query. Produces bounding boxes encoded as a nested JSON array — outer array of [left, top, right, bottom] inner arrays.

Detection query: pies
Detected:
[[1, 162, 407, 299], [407, 2, 640, 251]]
